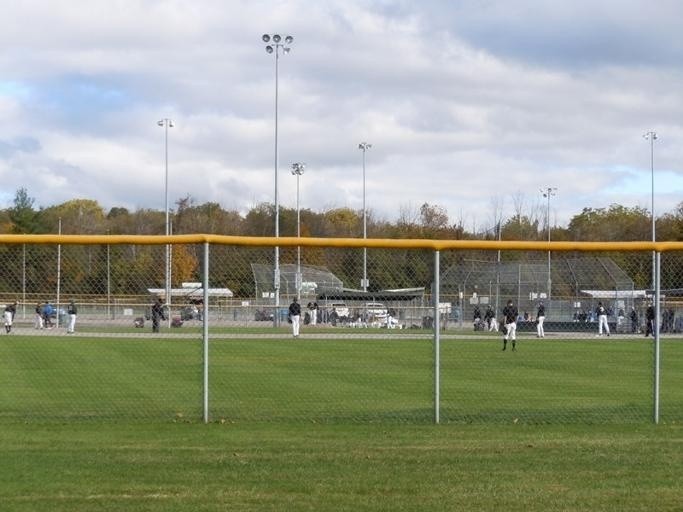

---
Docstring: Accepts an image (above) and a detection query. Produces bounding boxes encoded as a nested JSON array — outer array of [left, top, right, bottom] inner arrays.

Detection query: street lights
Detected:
[[643, 131, 658, 336], [288, 159, 307, 300], [156, 117, 177, 309], [539, 186, 559, 299], [263, 34, 293, 327], [358, 141, 372, 292]]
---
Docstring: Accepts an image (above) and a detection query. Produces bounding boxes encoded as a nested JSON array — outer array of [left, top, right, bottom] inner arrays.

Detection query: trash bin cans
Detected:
[[281, 310, 288, 320], [234, 308, 240, 320], [423, 317, 433, 328], [676, 317, 683, 332]]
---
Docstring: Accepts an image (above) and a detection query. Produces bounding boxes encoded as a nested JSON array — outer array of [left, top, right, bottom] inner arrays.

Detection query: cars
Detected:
[[255, 308, 274, 321], [320, 302, 388, 321]]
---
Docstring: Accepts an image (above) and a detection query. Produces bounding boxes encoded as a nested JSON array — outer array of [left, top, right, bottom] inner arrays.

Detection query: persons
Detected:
[[289, 297, 301, 337], [35, 301, 52, 329], [536, 300, 545, 337], [152, 299, 166, 333], [572, 302, 674, 336], [524, 311, 530, 322], [307, 300, 395, 329], [2, 301, 19, 333], [67, 301, 77, 333], [503, 299, 519, 352], [474, 304, 499, 332]]
